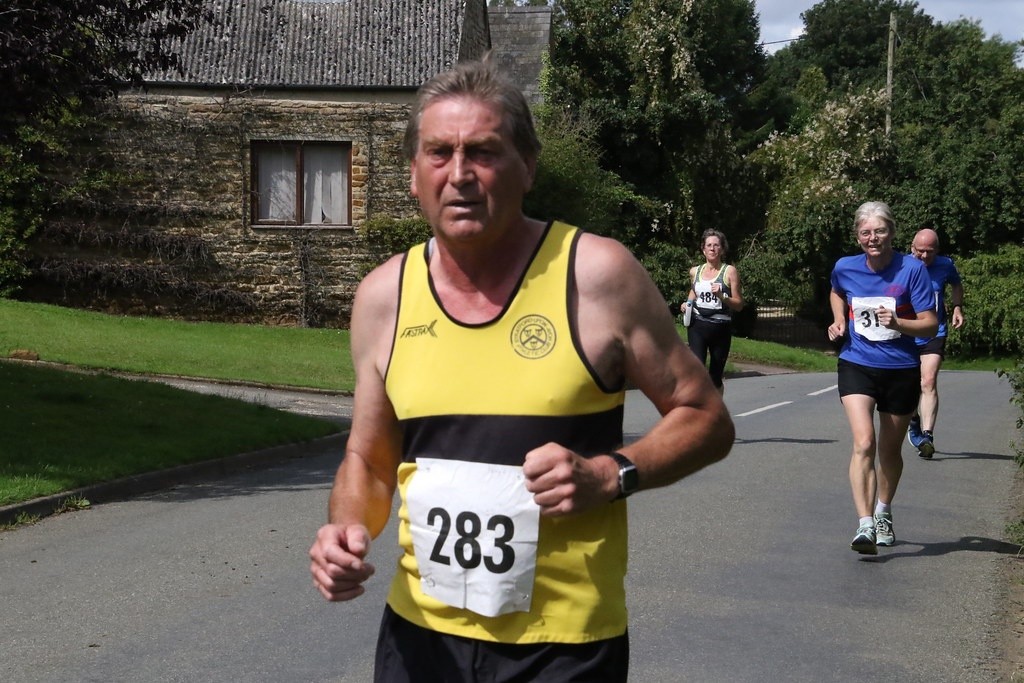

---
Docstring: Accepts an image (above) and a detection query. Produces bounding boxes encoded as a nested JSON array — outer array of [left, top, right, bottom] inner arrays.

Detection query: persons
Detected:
[[828, 201, 938, 555], [680, 228, 742, 400], [906, 230, 965, 459], [308, 57, 736, 683]]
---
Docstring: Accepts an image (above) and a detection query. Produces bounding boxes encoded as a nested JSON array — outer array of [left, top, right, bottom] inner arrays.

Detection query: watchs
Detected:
[[722, 292, 729, 303], [605, 451, 640, 502]]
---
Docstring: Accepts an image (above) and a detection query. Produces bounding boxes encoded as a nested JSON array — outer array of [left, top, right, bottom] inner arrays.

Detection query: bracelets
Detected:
[[687, 300, 694, 305], [953, 304, 962, 313]]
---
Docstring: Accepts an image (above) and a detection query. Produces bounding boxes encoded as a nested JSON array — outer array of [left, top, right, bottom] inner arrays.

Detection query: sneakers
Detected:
[[873, 512, 895, 546], [908, 416, 924, 447], [850, 521, 879, 555], [918, 437, 935, 458]]
